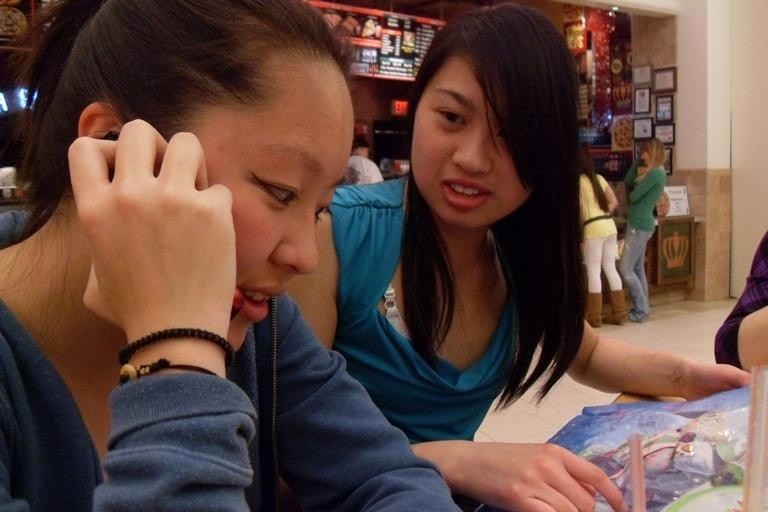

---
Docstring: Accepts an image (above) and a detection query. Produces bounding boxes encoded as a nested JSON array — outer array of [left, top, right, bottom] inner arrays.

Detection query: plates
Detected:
[[664, 483, 753, 512]]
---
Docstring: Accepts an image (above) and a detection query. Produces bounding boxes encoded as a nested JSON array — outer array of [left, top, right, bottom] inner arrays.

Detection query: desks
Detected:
[[532, 388, 767, 512]]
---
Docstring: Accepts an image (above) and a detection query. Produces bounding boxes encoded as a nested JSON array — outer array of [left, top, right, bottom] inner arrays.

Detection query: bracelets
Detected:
[[117, 358, 227, 389], [106, 326, 240, 366]]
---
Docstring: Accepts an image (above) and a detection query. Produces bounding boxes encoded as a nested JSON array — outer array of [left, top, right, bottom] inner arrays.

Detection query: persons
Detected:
[[714, 230, 767, 377], [575, 142, 628, 329], [1, 1, 461, 511], [617, 137, 665, 317], [279, 1, 755, 510], [350, 137, 371, 158]]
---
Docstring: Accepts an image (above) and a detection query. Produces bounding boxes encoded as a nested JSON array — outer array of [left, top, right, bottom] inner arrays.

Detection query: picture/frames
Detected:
[[632, 60, 678, 176]]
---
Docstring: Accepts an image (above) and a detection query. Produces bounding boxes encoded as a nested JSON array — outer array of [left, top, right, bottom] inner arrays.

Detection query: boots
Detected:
[[585, 293, 603, 328], [604, 290, 625, 323]]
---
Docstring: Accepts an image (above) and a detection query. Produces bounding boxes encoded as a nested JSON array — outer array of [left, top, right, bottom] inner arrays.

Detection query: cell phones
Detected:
[[103, 132, 245, 321]]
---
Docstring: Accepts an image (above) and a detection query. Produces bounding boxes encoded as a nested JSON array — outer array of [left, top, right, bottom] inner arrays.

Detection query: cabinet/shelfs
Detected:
[[312, 1, 448, 83]]
